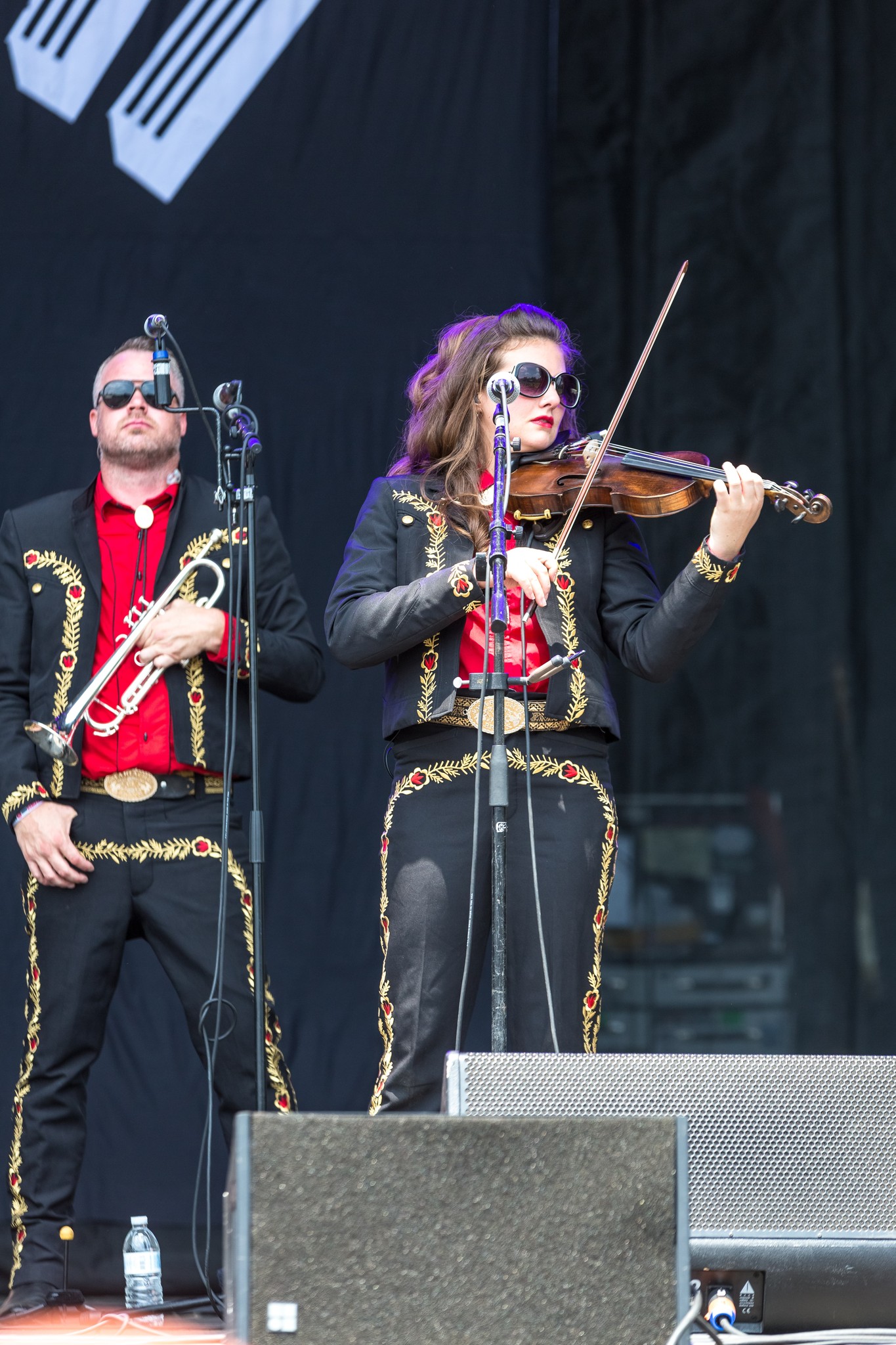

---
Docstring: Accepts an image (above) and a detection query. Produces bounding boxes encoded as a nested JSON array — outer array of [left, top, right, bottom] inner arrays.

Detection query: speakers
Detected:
[[441, 1048, 896, 1334], [216, 1109, 693, 1345]]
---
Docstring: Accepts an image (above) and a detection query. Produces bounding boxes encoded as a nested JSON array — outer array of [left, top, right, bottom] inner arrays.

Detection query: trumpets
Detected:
[[23, 528, 226, 766]]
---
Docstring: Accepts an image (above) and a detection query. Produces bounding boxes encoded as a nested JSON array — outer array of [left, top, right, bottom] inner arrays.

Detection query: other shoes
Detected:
[[0, 1287, 58, 1316]]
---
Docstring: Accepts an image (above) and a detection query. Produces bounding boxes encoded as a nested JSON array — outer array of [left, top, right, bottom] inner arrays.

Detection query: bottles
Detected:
[[123, 1216, 164, 1328]]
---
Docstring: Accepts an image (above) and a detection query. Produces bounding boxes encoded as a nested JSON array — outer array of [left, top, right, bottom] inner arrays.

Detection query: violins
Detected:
[[505, 440, 833, 524]]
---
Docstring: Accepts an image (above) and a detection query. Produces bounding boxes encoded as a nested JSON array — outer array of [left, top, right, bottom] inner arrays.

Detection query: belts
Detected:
[[427, 696, 572, 736], [81, 768, 233, 804]]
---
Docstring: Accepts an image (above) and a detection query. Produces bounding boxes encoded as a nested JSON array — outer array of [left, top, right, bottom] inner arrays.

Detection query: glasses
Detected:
[[481, 362, 582, 409], [96, 379, 181, 416]]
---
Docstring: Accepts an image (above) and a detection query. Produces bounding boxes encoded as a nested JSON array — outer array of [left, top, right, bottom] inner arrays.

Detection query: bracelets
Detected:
[[11, 800, 45, 829]]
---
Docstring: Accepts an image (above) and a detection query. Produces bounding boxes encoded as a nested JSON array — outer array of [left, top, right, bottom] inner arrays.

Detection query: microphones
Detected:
[[487, 371, 521, 405], [212, 378, 240, 409], [142, 314, 169, 340]]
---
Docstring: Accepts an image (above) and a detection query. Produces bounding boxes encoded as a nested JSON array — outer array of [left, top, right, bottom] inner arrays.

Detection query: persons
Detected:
[[324, 304, 765, 1117], [0, 336, 326, 1322]]
